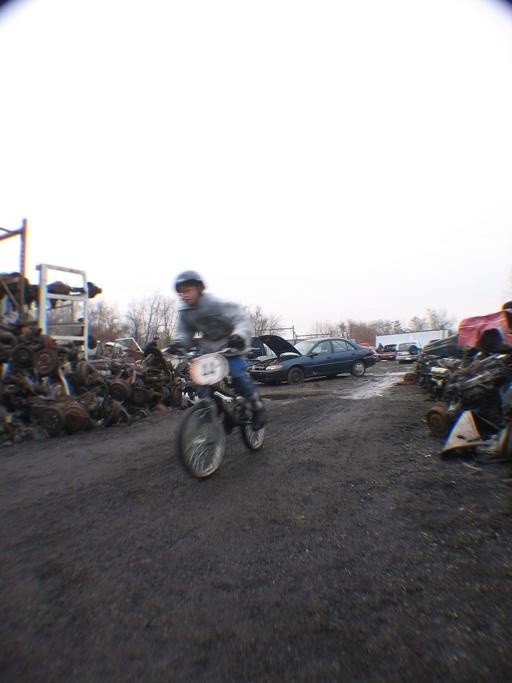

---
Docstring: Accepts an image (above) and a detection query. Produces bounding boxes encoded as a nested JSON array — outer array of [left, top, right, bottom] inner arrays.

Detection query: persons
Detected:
[[167, 271, 268, 431], [144, 336, 159, 356]]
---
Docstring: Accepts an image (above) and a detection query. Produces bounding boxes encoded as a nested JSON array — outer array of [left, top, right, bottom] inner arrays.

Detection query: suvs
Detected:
[[395, 340, 421, 363], [248, 336, 268, 358]]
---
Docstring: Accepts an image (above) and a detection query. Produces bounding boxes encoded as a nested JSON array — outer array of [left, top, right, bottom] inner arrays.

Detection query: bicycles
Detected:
[[163, 344, 268, 478]]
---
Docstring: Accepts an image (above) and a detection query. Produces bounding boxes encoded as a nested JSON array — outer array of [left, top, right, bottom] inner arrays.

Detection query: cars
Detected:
[[379, 343, 397, 359], [364, 346, 381, 361], [251, 333, 375, 384]]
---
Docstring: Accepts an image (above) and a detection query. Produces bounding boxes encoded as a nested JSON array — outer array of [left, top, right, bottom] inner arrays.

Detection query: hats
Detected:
[[175, 269, 205, 289]]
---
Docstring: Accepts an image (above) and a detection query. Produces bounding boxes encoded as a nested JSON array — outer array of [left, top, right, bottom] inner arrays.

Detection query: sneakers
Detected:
[[252, 400, 266, 430]]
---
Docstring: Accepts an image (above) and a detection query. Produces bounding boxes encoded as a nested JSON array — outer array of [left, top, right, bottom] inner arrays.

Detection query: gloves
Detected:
[[226, 334, 246, 351]]
